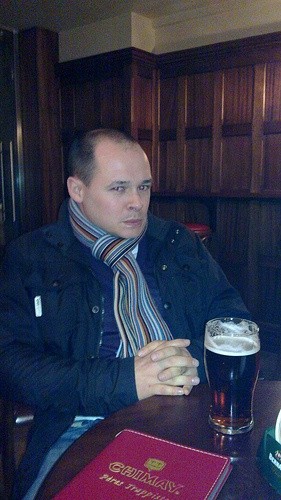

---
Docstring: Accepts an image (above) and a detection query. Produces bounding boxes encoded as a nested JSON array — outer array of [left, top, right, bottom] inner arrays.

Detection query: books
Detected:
[[52, 429, 232, 500]]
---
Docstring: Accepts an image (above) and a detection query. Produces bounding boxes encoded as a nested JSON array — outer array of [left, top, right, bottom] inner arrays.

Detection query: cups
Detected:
[[204, 319, 261, 433]]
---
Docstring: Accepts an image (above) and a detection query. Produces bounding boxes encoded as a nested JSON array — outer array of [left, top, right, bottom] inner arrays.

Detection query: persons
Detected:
[[0, 125, 253, 500]]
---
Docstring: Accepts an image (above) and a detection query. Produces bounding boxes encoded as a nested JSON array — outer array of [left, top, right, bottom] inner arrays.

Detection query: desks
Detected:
[[36, 380, 281, 500]]
